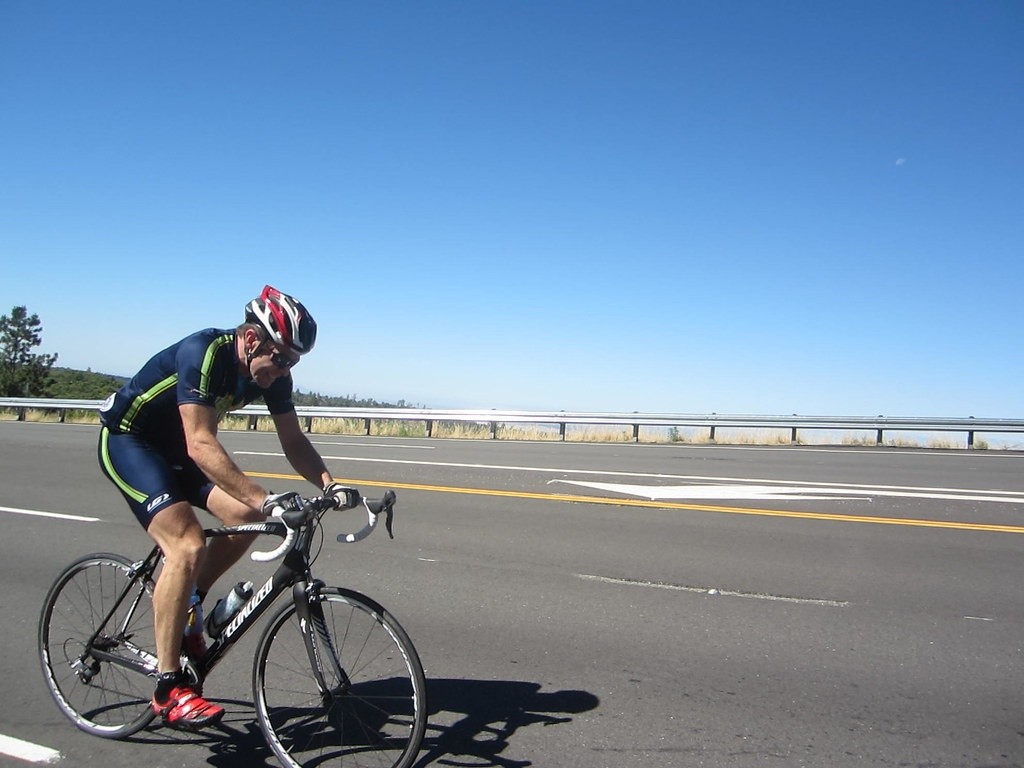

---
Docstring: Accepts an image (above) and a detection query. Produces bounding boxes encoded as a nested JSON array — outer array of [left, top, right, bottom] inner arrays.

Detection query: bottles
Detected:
[[202, 579, 254, 640]]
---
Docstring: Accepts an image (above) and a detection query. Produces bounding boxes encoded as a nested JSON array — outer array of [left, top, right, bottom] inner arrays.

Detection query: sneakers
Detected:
[[181, 607, 209, 666], [151, 677, 225, 730]]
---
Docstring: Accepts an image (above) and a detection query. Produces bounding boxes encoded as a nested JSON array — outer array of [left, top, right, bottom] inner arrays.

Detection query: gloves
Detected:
[[323, 482, 360, 511], [262, 491, 304, 516]]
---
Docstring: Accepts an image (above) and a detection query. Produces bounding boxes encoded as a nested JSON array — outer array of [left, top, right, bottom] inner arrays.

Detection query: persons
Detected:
[[95, 284, 360, 728]]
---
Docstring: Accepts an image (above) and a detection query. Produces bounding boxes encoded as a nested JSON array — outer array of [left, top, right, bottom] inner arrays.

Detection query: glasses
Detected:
[[259, 338, 300, 370]]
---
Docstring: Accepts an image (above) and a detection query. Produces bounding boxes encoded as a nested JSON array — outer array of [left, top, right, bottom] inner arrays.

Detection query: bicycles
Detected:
[[36, 479, 429, 768]]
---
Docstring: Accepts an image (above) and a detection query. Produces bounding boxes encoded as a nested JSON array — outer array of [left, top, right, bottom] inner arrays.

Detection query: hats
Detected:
[[245, 285, 317, 355]]
